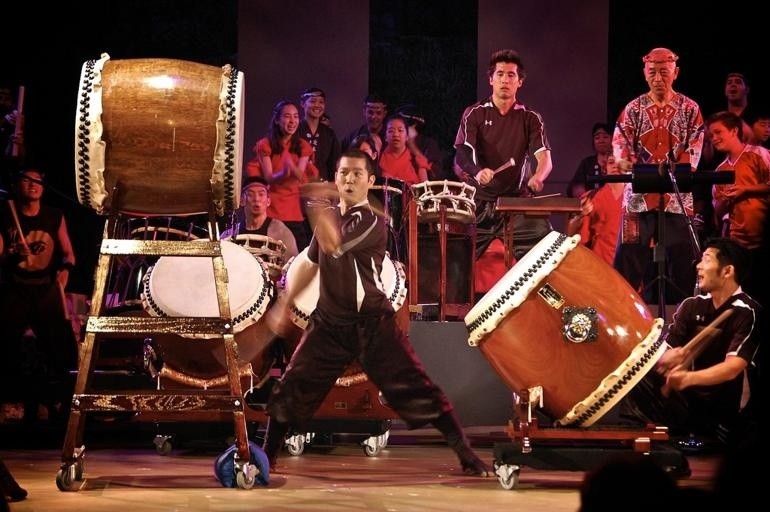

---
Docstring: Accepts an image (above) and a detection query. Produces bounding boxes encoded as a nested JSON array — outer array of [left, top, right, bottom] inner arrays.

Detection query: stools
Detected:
[[409, 208, 478, 322]]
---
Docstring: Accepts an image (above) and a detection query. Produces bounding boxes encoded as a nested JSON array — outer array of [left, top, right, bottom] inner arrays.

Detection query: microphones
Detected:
[[654, 149, 675, 176]]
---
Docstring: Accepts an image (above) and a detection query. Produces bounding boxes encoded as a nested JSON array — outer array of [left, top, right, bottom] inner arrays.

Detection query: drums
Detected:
[[296, 179, 406, 238], [279, 245, 408, 421], [464, 229, 667, 428], [74, 52, 245, 216], [223, 234, 287, 277], [408, 179, 481, 223], [130, 226, 197, 241], [141, 238, 274, 391]]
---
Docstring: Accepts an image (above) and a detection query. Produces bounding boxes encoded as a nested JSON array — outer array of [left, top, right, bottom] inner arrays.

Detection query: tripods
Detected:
[[634, 192, 689, 319]]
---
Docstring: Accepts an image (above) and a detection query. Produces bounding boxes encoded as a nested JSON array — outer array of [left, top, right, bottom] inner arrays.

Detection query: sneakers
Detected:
[[270, 464, 276, 474], [464, 458, 495, 478], [242, 374, 283, 411]]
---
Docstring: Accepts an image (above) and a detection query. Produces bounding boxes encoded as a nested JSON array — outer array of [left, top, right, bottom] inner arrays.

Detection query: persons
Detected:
[[294, 87, 340, 182], [566, 122, 613, 198], [751, 115, 770, 145], [611, 47, 705, 243], [0, 164, 78, 434], [633, 236, 763, 458], [252, 98, 315, 244], [708, 112, 770, 251], [394, 104, 443, 181], [376, 115, 433, 261], [340, 94, 388, 160], [453, 48, 553, 263], [567, 154, 627, 268], [245, 158, 262, 177], [220, 176, 299, 256], [723, 70, 749, 119], [350, 134, 379, 160], [256, 149, 495, 477]]
